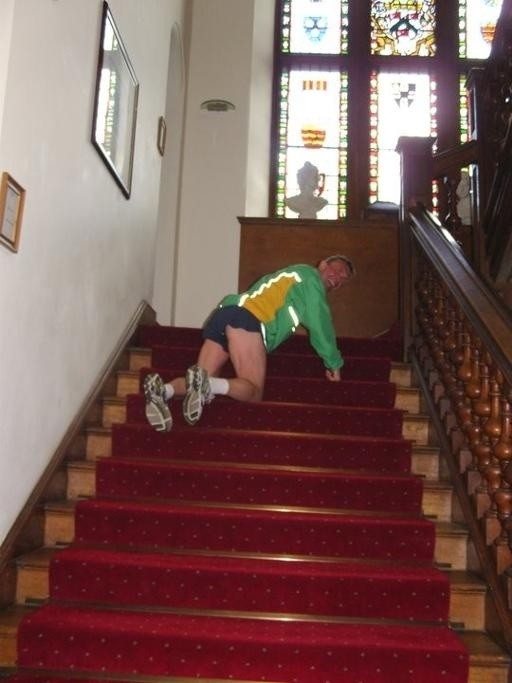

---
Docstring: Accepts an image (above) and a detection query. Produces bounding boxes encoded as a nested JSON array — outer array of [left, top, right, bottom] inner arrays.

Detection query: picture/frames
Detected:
[[0, 171, 27, 253], [89, 0, 142, 201]]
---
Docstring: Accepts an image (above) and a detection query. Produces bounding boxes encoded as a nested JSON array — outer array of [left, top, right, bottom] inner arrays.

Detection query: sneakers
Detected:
[[144, 372, 173, 432], [182, 365, 211, 426]]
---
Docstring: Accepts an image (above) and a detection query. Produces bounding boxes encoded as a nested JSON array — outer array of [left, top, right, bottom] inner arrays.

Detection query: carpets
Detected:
[[5, 311, 469, 683]]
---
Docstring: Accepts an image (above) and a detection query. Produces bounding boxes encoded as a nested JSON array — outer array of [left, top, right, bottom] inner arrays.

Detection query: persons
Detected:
[[143, 254, 356, 432]]
